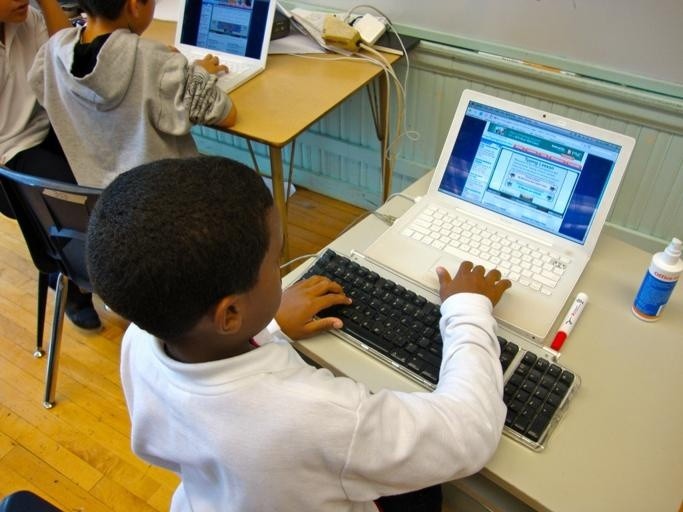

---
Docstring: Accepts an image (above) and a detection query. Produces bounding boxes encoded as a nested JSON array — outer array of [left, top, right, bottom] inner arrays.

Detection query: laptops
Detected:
[[365, 89, 636, 340], [174, 0, 276, 95]]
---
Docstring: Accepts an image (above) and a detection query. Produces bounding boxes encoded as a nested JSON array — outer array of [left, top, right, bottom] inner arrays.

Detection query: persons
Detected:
[[84, 155, 511, 512], [0, 1, 106, 337], [26, 0, 237, 189]]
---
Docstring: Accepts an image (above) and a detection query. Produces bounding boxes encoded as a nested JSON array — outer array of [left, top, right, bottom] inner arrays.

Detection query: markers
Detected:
[[550, 292, 589, 351]]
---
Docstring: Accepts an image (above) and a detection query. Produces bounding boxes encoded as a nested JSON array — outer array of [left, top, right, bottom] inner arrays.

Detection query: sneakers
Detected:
[[55, 285, 104, 331]]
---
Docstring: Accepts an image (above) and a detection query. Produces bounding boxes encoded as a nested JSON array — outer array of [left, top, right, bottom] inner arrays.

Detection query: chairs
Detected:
[[0, 490, 61, 512], [0, 168, 105, 412]]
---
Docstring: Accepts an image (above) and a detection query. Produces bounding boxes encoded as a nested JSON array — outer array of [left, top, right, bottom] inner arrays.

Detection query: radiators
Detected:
[[188, 1, 683, 260]]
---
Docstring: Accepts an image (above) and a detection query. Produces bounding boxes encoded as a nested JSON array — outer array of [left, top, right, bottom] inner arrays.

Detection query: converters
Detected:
[[354, 13, 385, 47]]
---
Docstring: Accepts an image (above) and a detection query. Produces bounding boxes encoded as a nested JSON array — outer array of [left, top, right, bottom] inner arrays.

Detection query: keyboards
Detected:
[[287, 249, 582, 453]]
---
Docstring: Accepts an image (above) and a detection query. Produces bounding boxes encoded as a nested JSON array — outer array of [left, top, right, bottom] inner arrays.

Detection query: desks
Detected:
[[280, 168, 683, 512], [139, 17, 418, 276]]
